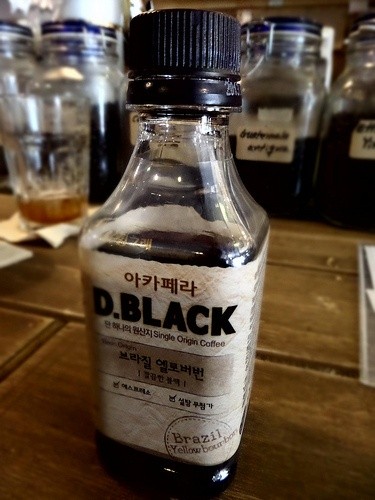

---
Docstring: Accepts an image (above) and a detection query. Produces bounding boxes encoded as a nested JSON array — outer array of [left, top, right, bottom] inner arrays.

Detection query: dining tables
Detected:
[[0, 199, 375, 500]]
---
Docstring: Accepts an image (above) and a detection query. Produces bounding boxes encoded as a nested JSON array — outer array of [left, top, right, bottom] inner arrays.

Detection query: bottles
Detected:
[[77, 6, 272, 500], [238, 10, 375, 233], [1, 18, 130, 207]]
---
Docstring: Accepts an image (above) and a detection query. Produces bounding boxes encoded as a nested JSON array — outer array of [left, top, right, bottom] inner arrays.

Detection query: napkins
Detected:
[[0, 204, 102, 250]]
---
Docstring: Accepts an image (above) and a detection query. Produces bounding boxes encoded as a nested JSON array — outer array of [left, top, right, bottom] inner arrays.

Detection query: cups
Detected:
[[1, 54, 90, 224]]
